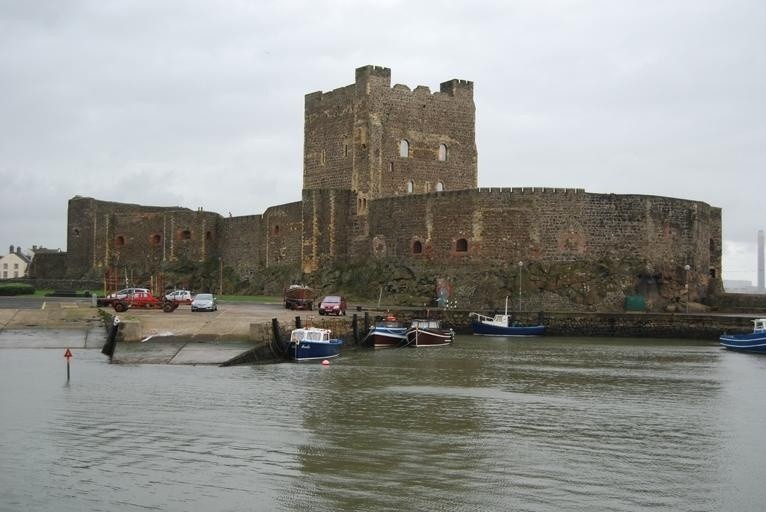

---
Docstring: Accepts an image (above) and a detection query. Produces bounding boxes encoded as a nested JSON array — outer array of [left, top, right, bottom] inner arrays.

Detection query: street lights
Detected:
[[684, 264, 692, 313], [517, 260, 524, 310]]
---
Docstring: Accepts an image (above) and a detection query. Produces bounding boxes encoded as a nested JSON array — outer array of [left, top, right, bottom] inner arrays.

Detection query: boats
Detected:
[[718, 317, 765, 353], [467, 310, 546, 338], [359, 314, 454, 348], [274, 323, 344, 361]]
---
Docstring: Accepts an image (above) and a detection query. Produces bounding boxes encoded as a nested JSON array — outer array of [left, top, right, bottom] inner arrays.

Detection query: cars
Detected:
[[191, 293, 218, 312], [165, 289, 193, 305], [317, 294, 347, 316], [106, 288, 151, 299]]
